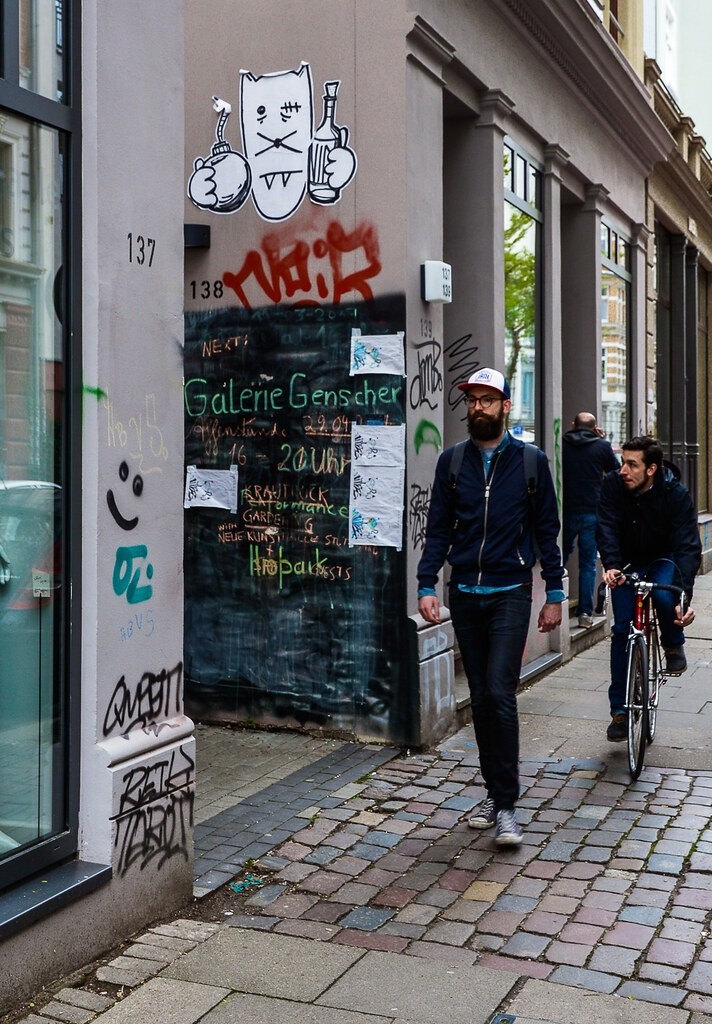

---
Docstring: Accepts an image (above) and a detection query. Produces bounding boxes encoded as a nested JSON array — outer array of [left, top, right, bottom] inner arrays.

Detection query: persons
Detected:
[[594, 436, 702, 743], [415, 368, 567, 848], [562, 412, 621, 629]]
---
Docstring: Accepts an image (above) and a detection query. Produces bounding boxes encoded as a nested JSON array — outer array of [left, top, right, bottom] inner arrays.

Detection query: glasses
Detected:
[[463, 393, 503, 408]]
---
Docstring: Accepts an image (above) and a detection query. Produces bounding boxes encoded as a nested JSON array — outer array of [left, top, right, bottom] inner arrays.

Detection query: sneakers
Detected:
[[468, 796, 499, 828], [493, 808, 524, 844], [664, 644, 688, 674], [606, 711, 628, 742], [577, 613, 594, 629]]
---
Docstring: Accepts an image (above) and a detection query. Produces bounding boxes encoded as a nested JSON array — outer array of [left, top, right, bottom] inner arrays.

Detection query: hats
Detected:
[[457, 367, 510, 399]]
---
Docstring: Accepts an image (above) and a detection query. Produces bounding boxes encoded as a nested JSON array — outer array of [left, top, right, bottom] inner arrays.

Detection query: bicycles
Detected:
[[594, 557, 689, 780]]
